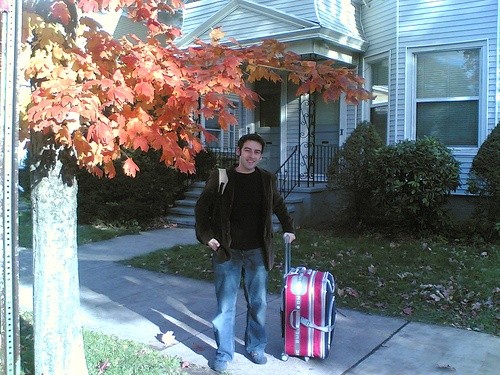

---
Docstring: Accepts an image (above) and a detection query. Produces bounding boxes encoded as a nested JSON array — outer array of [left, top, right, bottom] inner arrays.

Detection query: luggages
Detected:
[[280, 235, 338, 363]]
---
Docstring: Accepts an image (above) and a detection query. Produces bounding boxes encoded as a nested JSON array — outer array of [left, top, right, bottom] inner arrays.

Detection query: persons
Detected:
[[195, 134, 296, 372]]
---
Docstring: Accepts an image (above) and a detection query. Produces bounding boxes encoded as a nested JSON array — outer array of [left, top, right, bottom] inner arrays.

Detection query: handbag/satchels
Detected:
[[195, 219, 218, 246]]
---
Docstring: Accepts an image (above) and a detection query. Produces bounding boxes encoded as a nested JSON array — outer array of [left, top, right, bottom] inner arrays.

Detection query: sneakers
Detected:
[[212, 361, 229, 372], [247, 352, 267, 365]]
